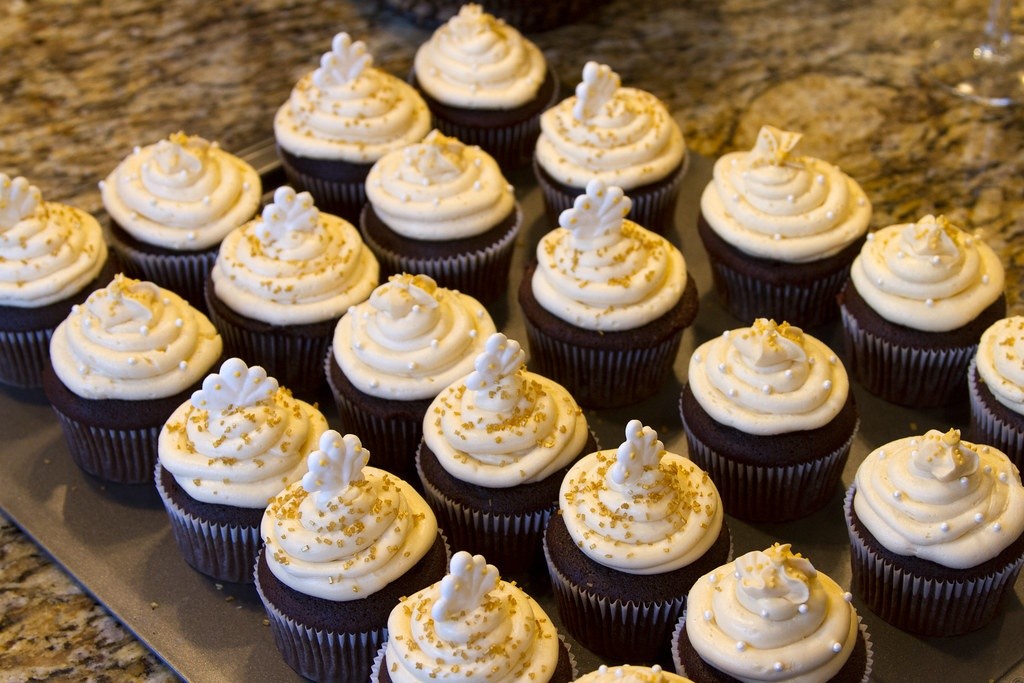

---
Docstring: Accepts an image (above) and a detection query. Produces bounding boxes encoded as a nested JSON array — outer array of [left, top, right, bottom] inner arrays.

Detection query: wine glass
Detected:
[[922, 1, 1024, 108]]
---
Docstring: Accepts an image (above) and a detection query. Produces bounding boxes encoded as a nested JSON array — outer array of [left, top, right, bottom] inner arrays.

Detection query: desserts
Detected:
[[0, 4, 1024, 683]]
